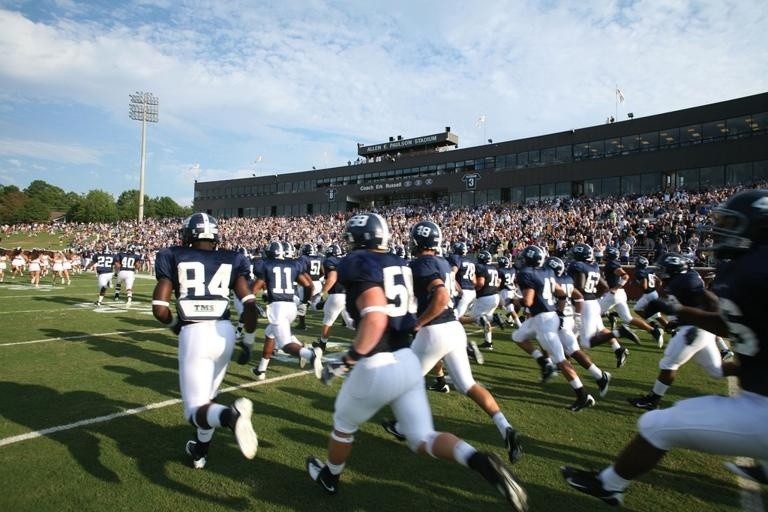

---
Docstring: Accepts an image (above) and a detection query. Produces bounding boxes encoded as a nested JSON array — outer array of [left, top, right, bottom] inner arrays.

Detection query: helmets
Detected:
[[656, 252, 688, 280], [603, 247, 620, 262], [447, 241, 469, 258], [126, 243, 136, 255], [571, 243, 594, 263], [342, 212, 390, 251], [102, 246, 112, 254], [694, 187, 768, 271], [179, 210, 222, 245], [521, 245, 544, 267], [238, 246, 251, 258], [634, 254, 649, 270], [476, 249, 494, 266], [546, 256, 564, 277], [407, 220, 443, 256], [496, 256, 510, 268], [265, 240, 344, 260]]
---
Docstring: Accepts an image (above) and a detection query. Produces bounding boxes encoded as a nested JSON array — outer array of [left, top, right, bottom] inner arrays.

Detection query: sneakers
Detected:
[[380, 417, 405, 441], [719, 348, 733, 361], [428, 382, 450, 394], [305, 454, 340, 495], [1, 270, 80, 288], [559, 465, 626, 510], [722, 459, 768, 485], [503, 426, 527, 465], [186, 441, 206, 470], [107, 280, 113, 289], [465, 295, 527, 366], [94, 301, 103, 306], [540, 310, 679, 415], [114, 296, 119, 301], [310, 341, 327, 380], [228, 397, 260, 461], [126, 297, 133, 306], [250, 367, 266, 380], [479, 451, 530, 512]]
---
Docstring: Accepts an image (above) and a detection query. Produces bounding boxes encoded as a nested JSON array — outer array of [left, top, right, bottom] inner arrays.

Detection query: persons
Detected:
[[1, 185, 768, 412], [151, 213, 257, 468], [305, 215, 528, 511], [559, 191, 768, 508]]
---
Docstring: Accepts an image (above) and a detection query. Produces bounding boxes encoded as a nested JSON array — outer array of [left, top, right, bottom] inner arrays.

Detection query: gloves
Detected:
[[320, 348, 362, 387], [233, 339, 255, 366]]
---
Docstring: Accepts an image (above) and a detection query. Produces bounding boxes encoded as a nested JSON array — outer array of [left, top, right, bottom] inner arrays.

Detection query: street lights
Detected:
[[127, 89, 162, 226]]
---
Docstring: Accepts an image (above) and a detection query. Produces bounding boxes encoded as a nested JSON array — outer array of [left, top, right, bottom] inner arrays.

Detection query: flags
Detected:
[[616, 88, 624, 104], [254, 155, 262, 163], [191, 163, 199, 171], [476, 114, 485, 127]]
